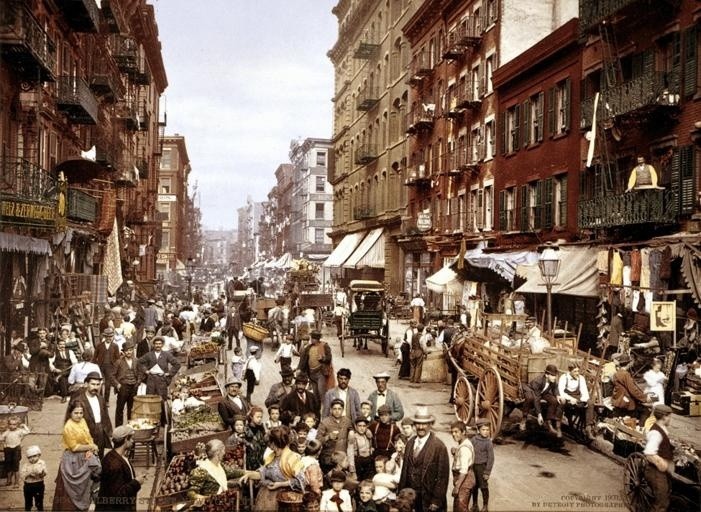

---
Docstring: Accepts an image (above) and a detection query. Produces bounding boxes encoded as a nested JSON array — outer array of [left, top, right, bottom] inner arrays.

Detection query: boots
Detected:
[[480, 488, 489, 512], [470, 488, 478, 510]]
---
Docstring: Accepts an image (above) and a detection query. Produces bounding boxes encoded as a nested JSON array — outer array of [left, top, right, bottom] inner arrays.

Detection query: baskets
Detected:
[[275, 475, 304, 512], [242, 320, 269, 343]]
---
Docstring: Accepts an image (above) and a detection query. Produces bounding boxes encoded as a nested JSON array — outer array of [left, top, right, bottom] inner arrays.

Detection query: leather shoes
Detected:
[[543, 421, 558, 434], [519, 416, 527, 431], [583, 428, 596, 440], [556, 426, 562, 438]]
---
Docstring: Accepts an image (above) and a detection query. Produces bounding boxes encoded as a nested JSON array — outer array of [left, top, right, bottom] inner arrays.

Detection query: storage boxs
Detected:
[[156, 455, 200, 505], [171, 420, 233, 452]]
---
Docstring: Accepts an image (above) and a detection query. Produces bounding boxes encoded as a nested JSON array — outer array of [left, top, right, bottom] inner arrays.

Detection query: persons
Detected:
[[0, 267, 701, 512], [625, 153, 658, 193]]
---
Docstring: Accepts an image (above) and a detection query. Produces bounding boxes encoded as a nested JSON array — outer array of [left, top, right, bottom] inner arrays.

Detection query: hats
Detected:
[[111, 425, 135, 440], [249, 345, 259, 353], [378, 405, 391, 415], [355, 416, 369, 424], [293, 375, 309, 382], [611, 352, 621, 359], [224, 377, 242, 389], [84, 372, 103, 384], [309, 330, 322, 337], [101, 326, 165, 352], [279, 364, 296, 376], [618, 354, 632, 363], [330, 470, 346, 482], [654, 405, 673, 415], [408, 409, 435, 423], [475, 417, 491, 427], [372, 372, 390, 378], [26, 447, 41, 458], [543, 365, 557, 376]]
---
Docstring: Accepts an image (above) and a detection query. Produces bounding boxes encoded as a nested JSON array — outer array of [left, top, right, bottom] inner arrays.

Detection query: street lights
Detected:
[[186, 254, 198, 304], [536, 239, 563, 336]]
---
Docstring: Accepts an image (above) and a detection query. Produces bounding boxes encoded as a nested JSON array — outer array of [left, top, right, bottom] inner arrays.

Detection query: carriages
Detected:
[[336, 278, 392, 359], [439, 309, 609, 445]]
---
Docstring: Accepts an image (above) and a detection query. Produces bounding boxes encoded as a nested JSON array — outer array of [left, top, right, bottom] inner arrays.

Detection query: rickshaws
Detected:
[[622, 447, 701, 512]]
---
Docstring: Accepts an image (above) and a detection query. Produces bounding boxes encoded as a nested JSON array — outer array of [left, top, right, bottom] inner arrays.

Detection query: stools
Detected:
[[126, 436, 155, 468]]
